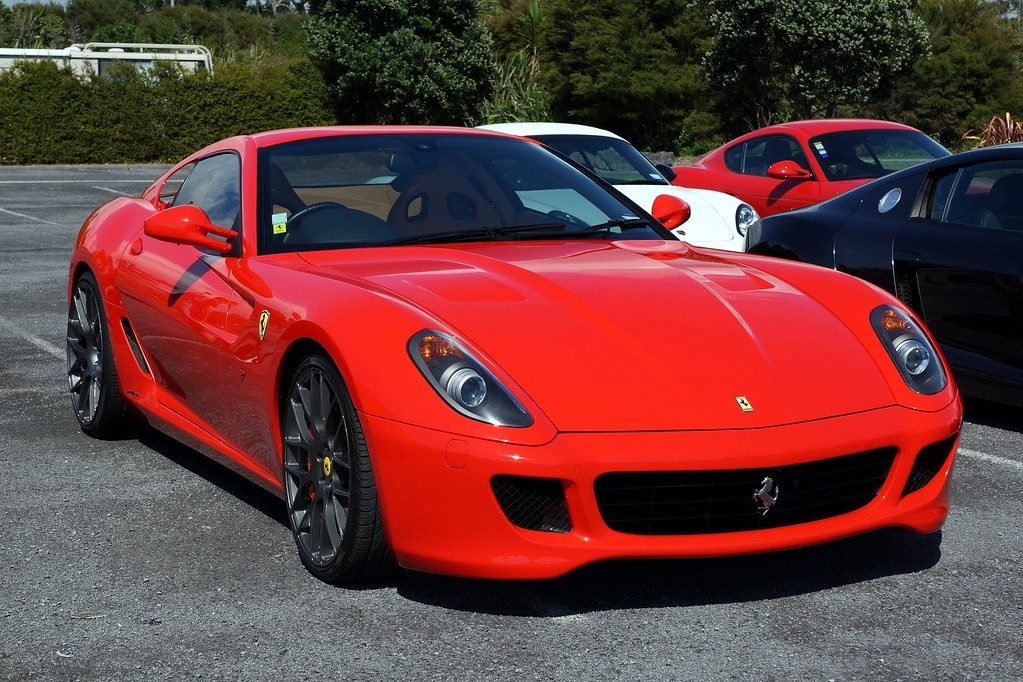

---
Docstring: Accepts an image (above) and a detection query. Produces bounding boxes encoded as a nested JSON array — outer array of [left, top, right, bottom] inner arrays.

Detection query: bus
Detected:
[[0, 43, 218, 91]]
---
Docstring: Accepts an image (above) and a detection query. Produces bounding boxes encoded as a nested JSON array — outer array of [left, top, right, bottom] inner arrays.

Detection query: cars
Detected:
[[747, 137, 1022, 431]]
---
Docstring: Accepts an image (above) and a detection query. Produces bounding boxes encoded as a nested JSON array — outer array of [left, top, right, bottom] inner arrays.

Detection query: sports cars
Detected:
[[64, 123, 968, 591], [367, 122, 765, 259], [664, 118, 1004, 226]]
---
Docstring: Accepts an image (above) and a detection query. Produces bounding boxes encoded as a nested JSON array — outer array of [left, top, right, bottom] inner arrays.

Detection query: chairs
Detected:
[[387, 166, 504, 239], [760, 140, 792, 176], [268, 163, 307, 214], [826, 138, 870, 168], [978, 174, 1023, 229]]
